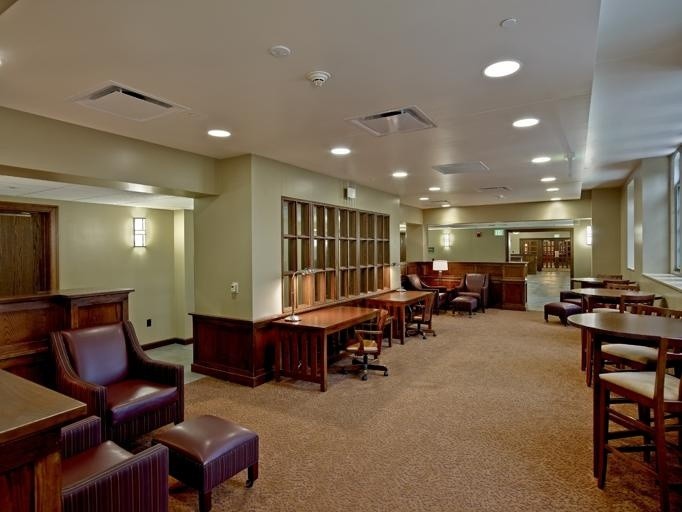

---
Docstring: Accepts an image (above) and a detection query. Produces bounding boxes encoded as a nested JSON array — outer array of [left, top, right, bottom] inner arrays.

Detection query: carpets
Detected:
[[133, 308, 679, 511]]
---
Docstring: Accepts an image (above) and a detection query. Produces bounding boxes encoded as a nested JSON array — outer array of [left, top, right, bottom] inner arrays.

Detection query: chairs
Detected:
[[540, 273, 682, 512], [337, 310, 390, 383], [404, 291, 440, 339]]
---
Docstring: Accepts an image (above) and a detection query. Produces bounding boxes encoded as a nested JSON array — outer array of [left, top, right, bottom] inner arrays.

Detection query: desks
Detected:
[[273, 290, 382, 392], [370, 289, 434, 346], [0, 363, 88, 511]]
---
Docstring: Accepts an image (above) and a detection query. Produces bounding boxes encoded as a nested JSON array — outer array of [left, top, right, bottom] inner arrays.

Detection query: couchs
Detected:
[[51, 319, 183, 445], [152, 414, 259, 512], [40, 416, 170, 511], [402, 274, 447, 316], [451, 295, 479, 314], [454, 272, 490, 313]]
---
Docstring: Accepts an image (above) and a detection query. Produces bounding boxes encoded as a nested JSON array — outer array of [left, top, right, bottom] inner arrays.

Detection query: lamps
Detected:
[[585, 224, 592, 246], [432, 260, 449, 285], [284, 264, 310, 322], [442, 232, 451, 249], [131, 217, 148, 248]]
